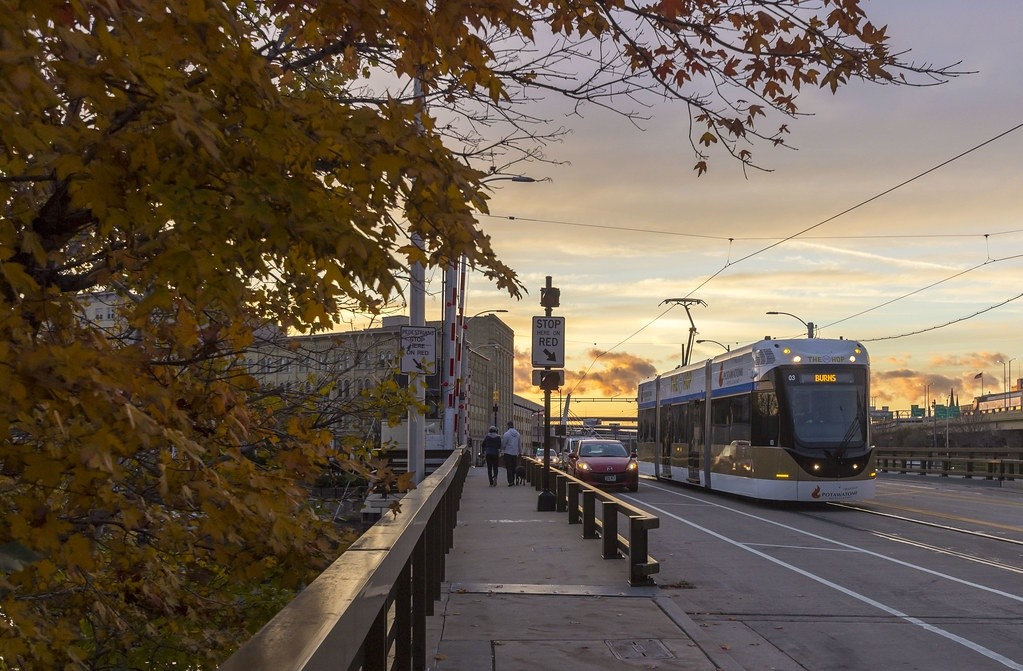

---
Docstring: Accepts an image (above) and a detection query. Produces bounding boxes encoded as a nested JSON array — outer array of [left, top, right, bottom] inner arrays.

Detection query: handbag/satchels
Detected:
[[498, 451, 504, 457]]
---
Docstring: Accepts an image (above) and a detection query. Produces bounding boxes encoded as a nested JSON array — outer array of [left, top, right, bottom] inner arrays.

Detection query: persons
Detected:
[[501, 422, 522, 487], [482, 426, 501, 486]]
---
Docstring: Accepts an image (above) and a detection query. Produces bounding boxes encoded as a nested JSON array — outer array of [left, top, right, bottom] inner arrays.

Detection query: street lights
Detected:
[[919, 384, 926, 408], [1009, 358, 1017, 407], [444, 177, 536, 446], [696, 340, 730, 352], [997, 361, 1006, 408], [928, 383, 934, 407], [767, 312, 814, 339]]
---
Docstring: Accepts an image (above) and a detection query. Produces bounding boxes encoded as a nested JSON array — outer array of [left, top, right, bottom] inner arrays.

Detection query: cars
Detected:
[[536, 448, 560, 470], [566, 439, 639, 492], [561, 437, 597, 470]]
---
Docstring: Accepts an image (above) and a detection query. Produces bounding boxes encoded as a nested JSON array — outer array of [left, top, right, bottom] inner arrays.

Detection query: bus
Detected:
[[637, 340, 876, 504]]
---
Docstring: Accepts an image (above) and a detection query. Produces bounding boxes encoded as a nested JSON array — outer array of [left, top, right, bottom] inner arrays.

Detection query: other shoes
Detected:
[[508, 482, 515, 486], [493, 478, 497, 486], [489, 479, 493, 484]]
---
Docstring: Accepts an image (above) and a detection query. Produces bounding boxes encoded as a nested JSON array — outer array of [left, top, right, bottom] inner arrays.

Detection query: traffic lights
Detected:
[[541, 371, 560, 390], [540, 288, 560, 306]]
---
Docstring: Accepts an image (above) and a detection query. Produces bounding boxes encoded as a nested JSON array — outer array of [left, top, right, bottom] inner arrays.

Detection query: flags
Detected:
[[974, 373, 982, 379]]
